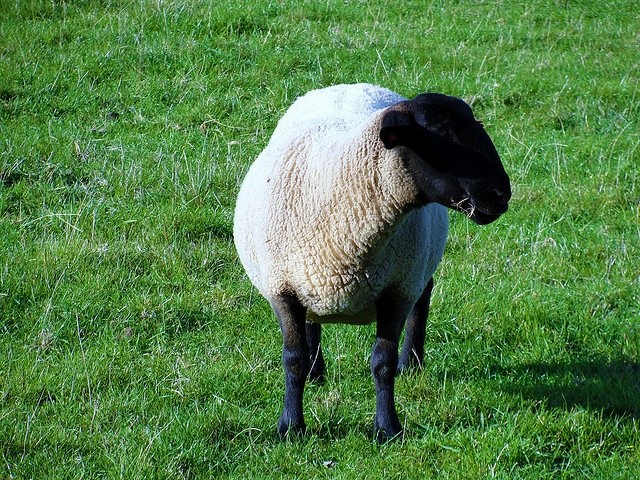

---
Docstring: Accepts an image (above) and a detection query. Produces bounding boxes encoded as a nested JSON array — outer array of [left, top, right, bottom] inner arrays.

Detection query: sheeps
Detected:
[[231, 81, 512, 444]]
